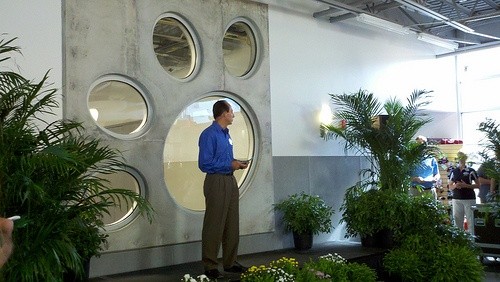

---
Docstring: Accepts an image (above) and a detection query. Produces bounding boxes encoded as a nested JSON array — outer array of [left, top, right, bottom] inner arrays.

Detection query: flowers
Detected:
[[181, 273, 216, 282], [241, 253, 350, 282]]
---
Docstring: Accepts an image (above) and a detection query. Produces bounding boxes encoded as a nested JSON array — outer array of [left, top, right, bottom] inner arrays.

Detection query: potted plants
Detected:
[[62, 222, 109, 282], [273, 191, 336, 250], [319, 89, 442, 249]]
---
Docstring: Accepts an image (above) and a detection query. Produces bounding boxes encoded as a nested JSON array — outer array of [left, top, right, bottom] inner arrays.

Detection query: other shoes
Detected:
[[204, 269, 221, 279], [224, 264, 251, 273]]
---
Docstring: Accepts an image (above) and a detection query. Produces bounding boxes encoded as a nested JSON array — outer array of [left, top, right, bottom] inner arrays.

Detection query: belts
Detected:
[[411, 186, 434, 190]]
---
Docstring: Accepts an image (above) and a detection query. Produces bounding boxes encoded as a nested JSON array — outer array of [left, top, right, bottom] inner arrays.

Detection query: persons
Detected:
[[0, 216, 14, 268], [448, 151, 477, 247], [408, 136, 441, 206], [477, 162, 499, 205], [198, 100, 252, 280]]
